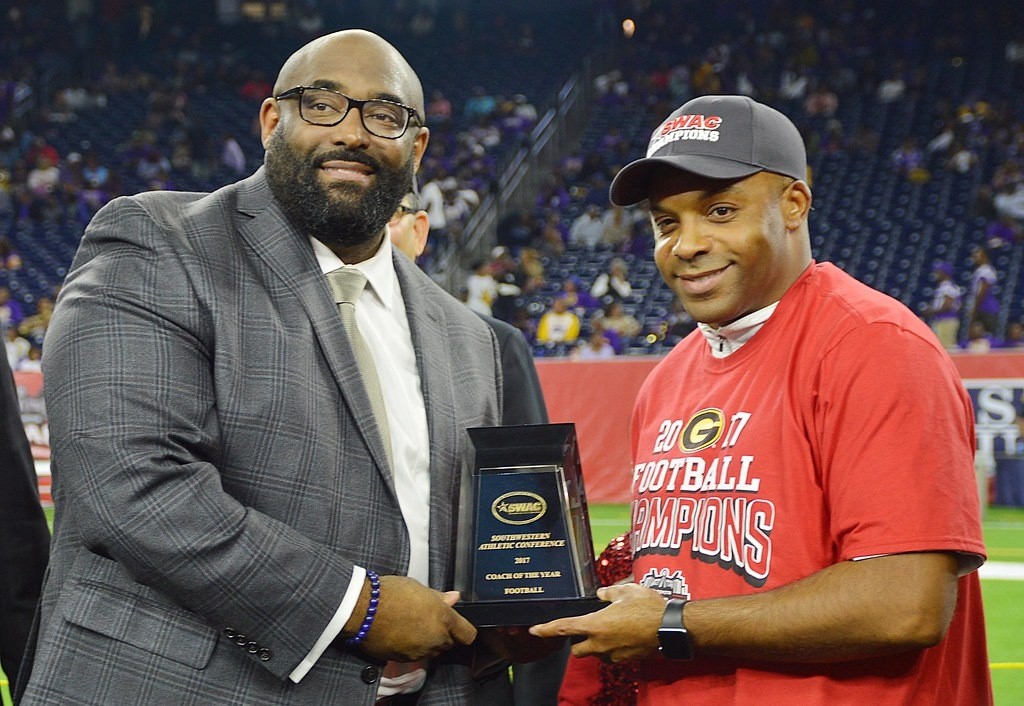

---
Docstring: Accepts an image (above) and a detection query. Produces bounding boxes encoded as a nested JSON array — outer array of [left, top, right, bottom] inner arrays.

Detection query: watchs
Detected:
[[657, 599, 692, 662]]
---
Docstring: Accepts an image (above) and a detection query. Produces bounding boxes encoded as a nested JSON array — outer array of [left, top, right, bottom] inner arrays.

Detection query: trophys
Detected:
[[452, 422, 611, 627]]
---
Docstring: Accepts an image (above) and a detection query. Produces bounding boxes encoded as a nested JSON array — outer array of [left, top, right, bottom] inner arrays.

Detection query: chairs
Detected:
[[0, 0, 1024, 359]]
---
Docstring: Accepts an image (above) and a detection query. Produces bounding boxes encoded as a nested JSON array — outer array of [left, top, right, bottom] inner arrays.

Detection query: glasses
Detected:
[[275, 85, 423, 139]]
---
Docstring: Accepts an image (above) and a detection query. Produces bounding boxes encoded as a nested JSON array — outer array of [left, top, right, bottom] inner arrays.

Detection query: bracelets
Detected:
[[344, 571, 380, 644]]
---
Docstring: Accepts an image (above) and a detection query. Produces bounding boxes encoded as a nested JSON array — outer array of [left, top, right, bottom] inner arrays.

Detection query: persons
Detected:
[[0, 30, 503, 706], [386, 176, 570, 706], [0, 0, 1024, 373], [529, 95, 994, 706]]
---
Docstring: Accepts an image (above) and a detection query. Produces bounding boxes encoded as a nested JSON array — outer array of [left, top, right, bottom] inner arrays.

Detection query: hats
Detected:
[[609, 95, 809, 208], [491, 246, 505, 259]]
